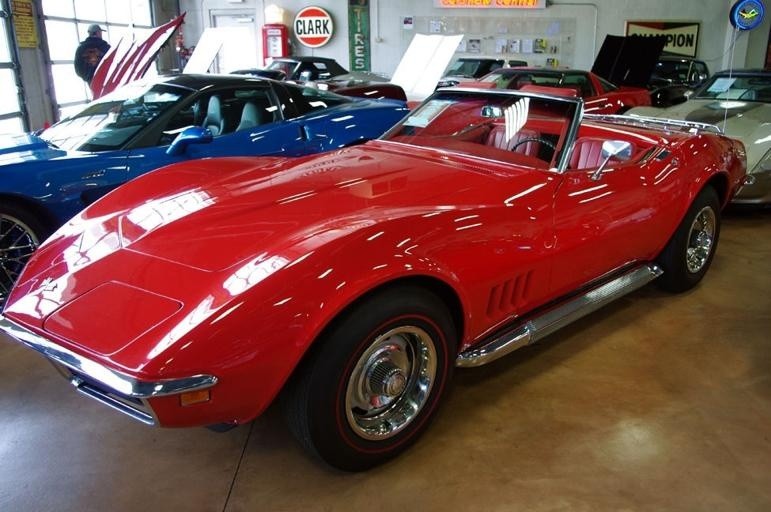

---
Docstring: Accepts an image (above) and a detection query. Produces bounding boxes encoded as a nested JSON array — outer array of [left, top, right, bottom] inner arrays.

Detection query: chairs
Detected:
[[483, 124, 620, 169], [197, 94, 263, 138]]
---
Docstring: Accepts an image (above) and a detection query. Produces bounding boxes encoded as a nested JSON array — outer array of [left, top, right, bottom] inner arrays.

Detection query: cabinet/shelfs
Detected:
[[399, 16, 577, 67]]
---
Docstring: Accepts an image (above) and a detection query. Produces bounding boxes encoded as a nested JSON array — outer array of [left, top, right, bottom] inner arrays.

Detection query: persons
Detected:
[[72, 24, 113, 87]]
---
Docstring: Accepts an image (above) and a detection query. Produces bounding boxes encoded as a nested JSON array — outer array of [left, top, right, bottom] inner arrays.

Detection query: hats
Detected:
[[88, 24, 106, 32]]
